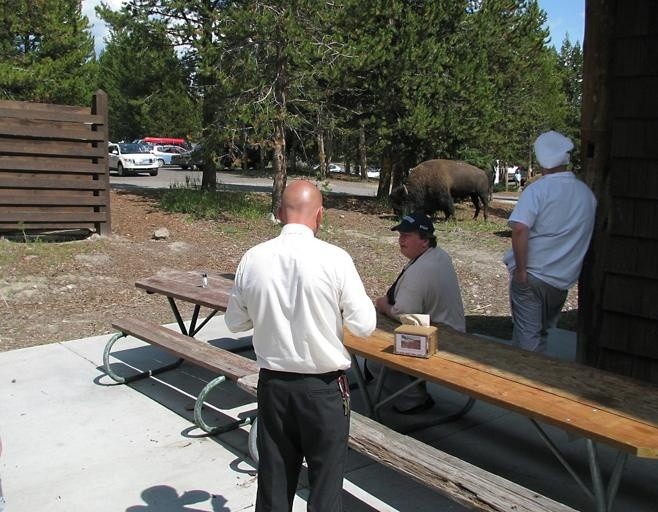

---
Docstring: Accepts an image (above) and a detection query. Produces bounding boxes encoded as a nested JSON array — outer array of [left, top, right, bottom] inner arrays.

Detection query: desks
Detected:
[[136, 268, 658, 459]]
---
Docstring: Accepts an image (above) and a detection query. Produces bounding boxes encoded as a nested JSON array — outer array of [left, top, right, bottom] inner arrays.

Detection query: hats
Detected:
[[392, 214, 434, 234], [534, 130, 573, 169]]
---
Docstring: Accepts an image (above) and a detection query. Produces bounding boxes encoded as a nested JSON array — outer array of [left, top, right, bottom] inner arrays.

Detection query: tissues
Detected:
[[393, 313, 438, 358]]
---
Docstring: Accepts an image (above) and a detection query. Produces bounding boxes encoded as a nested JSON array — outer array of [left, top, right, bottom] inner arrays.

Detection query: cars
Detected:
[[308, 157, 529, 188], [105, 134, 205, 178]]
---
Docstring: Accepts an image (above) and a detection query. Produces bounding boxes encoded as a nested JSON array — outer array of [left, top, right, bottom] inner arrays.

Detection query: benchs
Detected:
[[235, 371, 577, 512], [102, 311, 262, 428]]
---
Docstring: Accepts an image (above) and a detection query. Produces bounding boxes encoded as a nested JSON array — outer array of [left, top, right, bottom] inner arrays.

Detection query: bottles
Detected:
[[202, 274, 209, 288]]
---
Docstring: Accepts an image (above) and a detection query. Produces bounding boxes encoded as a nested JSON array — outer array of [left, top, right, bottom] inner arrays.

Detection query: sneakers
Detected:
[[393, 394, 435, 415]]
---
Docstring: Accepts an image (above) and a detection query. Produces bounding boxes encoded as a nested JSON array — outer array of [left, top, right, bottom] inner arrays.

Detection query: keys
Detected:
[[337, 374, 351, 416]]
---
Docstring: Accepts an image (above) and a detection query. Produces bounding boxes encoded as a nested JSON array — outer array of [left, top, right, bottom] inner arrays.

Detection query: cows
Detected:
[[387, 159, 493, 222]]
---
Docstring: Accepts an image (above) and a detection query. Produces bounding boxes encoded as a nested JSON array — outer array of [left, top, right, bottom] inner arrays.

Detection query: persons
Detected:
[[513, 170, 522, 189], [223, 177, 380, 511], [363, 212, 467, 417], [502, 128, 599, 356]]
